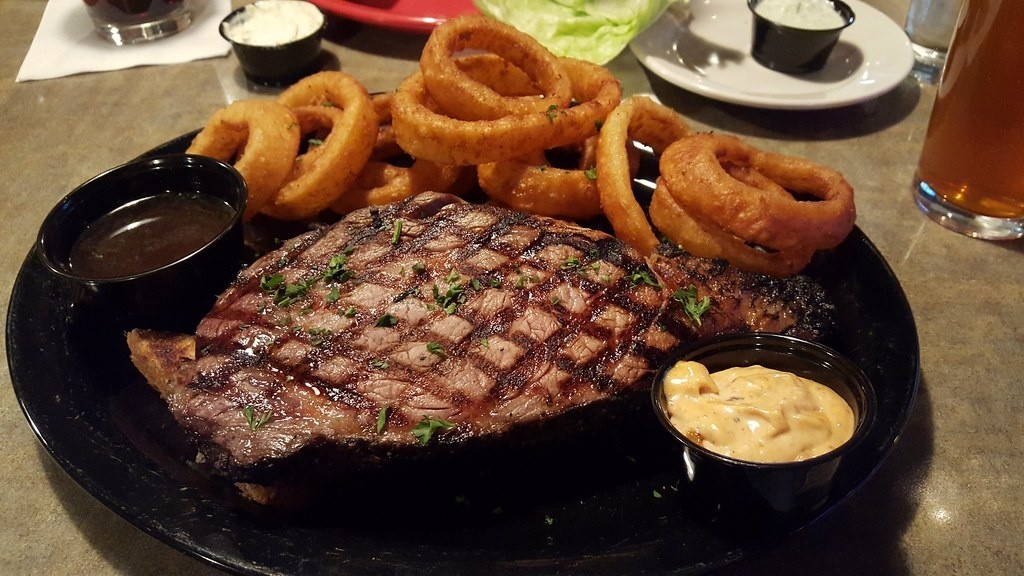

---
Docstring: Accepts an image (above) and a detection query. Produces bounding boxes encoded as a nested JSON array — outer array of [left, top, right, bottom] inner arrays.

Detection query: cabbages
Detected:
[[473, 0, 678, 67]]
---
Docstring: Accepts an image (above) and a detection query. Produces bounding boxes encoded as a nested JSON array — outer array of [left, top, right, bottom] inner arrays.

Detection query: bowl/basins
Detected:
[[37, 153, 248, 326], [746, 0, 856, 75], [650, 332, 877, 522], [218, 1, 328, 88]]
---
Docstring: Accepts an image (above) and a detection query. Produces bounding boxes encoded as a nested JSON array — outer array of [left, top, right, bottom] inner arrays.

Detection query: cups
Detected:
[[81, 0, 192, 45], [911, 0, 1024, 240], [905, 0, 964, 82]]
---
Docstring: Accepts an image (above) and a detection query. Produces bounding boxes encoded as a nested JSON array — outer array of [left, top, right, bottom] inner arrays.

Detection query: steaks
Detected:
[[126, 191, 838, 504]]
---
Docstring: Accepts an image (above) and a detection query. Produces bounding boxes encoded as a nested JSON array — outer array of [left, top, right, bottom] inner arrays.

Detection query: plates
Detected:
[[310, 0, 486, 36], [628, 0, 915, 111], [5, 125, 921, 576]]
